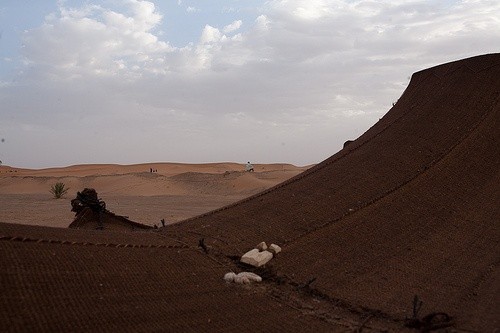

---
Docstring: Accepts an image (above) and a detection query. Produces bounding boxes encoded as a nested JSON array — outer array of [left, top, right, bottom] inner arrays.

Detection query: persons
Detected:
[[247, 161, 255, 172]]
